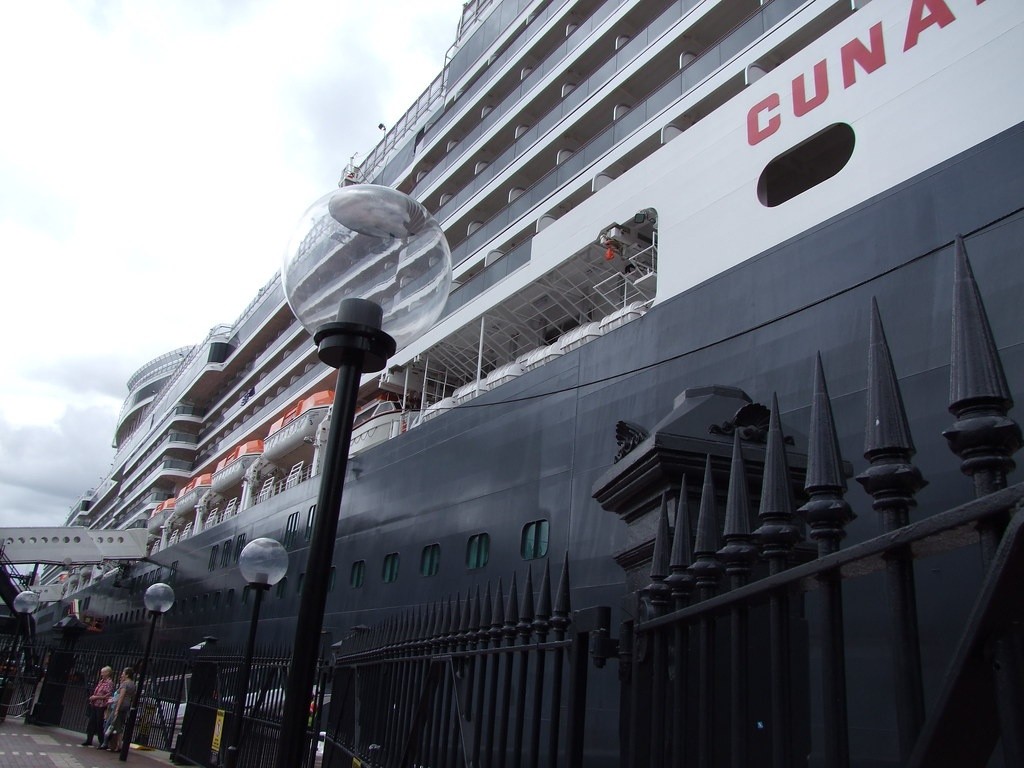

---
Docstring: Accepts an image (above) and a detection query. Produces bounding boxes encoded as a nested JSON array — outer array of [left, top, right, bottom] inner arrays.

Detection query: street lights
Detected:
[[120, 582, 176, 761], [222, 537, 289, 768], [0, 591, 39, 722], [273, 182, 453, 768]]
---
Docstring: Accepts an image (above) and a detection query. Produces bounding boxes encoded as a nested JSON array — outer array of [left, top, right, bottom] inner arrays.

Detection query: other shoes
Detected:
[[107, 746, 118, 751], [82, 741, 92, 746], [97, 744, 108, 750]]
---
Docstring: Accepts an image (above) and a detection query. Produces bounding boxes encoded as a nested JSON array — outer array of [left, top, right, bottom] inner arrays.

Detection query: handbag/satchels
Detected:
[[86, 703, 92, 717], [103, 718, 123, 741]]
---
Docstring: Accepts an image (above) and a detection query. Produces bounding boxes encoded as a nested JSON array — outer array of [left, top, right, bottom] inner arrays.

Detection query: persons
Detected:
[[81, 666, 134, 752], [307, 685, 320, 729]]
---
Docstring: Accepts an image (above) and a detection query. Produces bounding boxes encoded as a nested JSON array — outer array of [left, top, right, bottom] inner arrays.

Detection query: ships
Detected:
[[38, 0, 1024, 768]]
